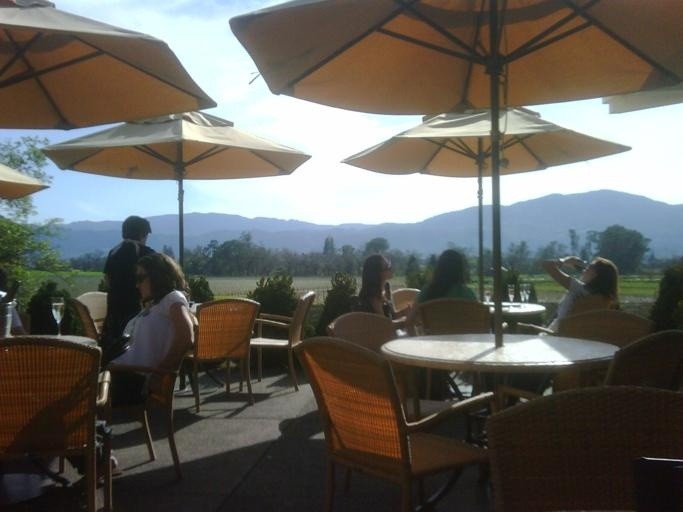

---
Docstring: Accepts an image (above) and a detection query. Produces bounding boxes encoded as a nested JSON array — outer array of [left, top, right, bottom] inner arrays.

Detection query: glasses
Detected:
[[136, 273, 147, 284]]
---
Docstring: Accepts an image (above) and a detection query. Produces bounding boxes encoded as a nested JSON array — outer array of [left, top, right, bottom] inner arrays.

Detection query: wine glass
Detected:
[[48, 293, 67, 339], [482, 285, 493, 306], [506, 280, 532, 311]]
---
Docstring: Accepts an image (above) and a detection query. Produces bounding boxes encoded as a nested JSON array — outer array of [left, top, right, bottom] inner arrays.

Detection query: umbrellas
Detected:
[[348, 106, 635, 310], [38, 105, 316, 267], [229, 0, 682, 413], [0, 1, 215, 135]]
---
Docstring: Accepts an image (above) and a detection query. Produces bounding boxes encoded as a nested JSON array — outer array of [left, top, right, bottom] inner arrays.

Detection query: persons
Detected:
[[542, 255, 618, 336], [0, 270, 26, 337], [358, 255, 407, 330], [649, 267, 683, 332], [415, 249, 478, 304], [100, 215, 157, 360], [109, 250, 199, 405], [29, 283, 58, 335], [0, 162, 51, 200]]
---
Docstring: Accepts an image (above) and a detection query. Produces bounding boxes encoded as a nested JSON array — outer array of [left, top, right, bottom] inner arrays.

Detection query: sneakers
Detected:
[[97, 457, 117, 483]]
